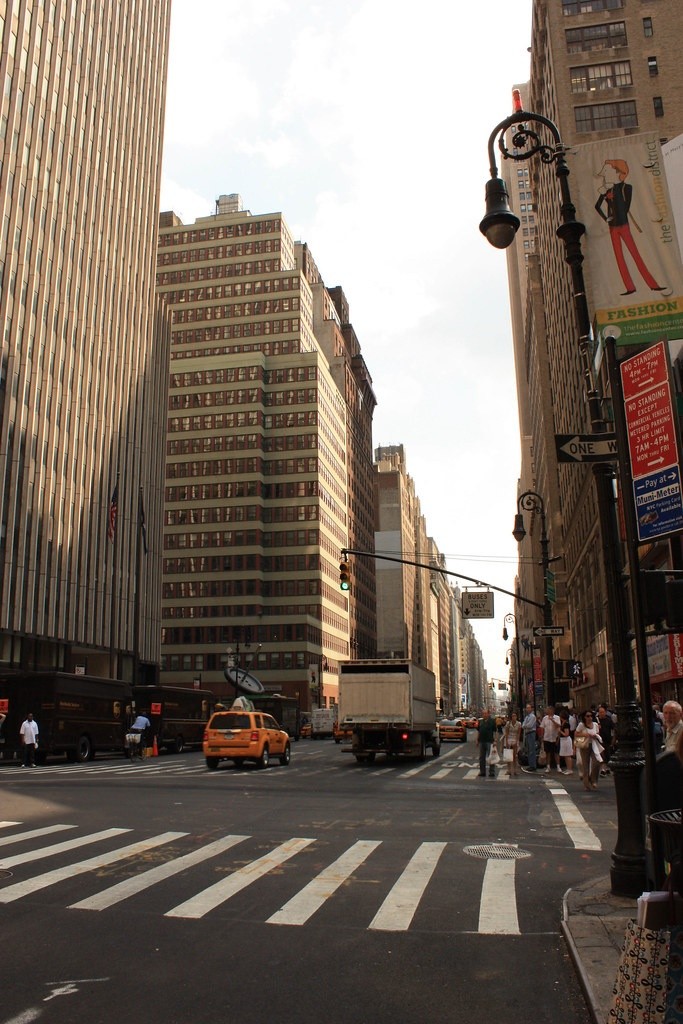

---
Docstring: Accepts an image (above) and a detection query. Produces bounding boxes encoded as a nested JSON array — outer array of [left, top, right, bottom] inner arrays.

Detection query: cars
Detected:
[[439, 719, 467, 743], [462, 717, 478, 728], [203, 711, 291, 770], [333, 719, 353, 745], [301, 722, 311, 739]]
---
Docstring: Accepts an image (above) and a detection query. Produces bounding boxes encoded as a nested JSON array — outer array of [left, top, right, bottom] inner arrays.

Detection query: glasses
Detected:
[[585, 714, 593, 717]]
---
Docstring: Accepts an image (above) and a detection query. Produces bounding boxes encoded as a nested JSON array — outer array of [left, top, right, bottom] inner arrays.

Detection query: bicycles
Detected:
[[129, 732, 148, 763]]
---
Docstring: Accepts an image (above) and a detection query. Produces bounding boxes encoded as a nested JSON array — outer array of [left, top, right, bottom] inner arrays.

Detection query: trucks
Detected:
[[311, 710, 336, 740], [336, 659, 441, 764]]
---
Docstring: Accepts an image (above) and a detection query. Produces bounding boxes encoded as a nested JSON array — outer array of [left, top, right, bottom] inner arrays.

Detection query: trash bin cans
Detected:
[[647, 808, 683, 894]]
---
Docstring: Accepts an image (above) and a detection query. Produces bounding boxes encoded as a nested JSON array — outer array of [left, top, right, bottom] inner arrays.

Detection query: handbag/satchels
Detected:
[[537, 740, 546, 765], [574, 722, 589, 749], [503, 745, 513, 763], [488, 744, 501, 765]]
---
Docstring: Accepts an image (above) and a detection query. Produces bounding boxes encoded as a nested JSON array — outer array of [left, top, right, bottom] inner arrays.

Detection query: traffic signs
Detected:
[[461, 592, 494, 619], [553, 434, 620, 463], [531, 626, 565, 637]]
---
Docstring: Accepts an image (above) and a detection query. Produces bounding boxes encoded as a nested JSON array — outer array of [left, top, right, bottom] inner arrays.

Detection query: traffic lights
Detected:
[[566, 661, 583, 678], [340, 562, 350, 591], [488, 683, 492, 690]]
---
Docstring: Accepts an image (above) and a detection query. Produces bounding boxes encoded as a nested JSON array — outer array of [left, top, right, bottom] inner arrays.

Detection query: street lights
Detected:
[[480, 111, 662, 897], [505, 648, 519, 721], [512, 491, 556, 710], [503, 613, 524, 742]]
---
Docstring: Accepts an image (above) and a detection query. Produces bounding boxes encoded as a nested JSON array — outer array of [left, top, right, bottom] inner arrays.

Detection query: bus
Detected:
[[132, 685, 217, 755], [5, 670, 132, 764]]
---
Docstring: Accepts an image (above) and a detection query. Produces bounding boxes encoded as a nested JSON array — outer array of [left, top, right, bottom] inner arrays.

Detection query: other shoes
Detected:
[[478, 773, 485, 776], [490, 773, 495, 777], [505, 772, 511, 775], [514, 772, 520, 775], [30, 763, 36, 767], [600, 770, 606, 777], [526, 767, 537, 771], [592, 781, 598, 788], [606, 769, 611, 776], [557, 767, 562, 772], [545, 769, 551, 773], [584, 785, 589, 790], [562, 769, 573, 775], [22, 764, 25, 768], [138, 755, 143, 758]]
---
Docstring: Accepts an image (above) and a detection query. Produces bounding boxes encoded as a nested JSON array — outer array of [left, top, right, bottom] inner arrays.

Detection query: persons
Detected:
[[130, 712, 151, 760], [475, 700, 683, 791], [19, 713, 39, 769]]
